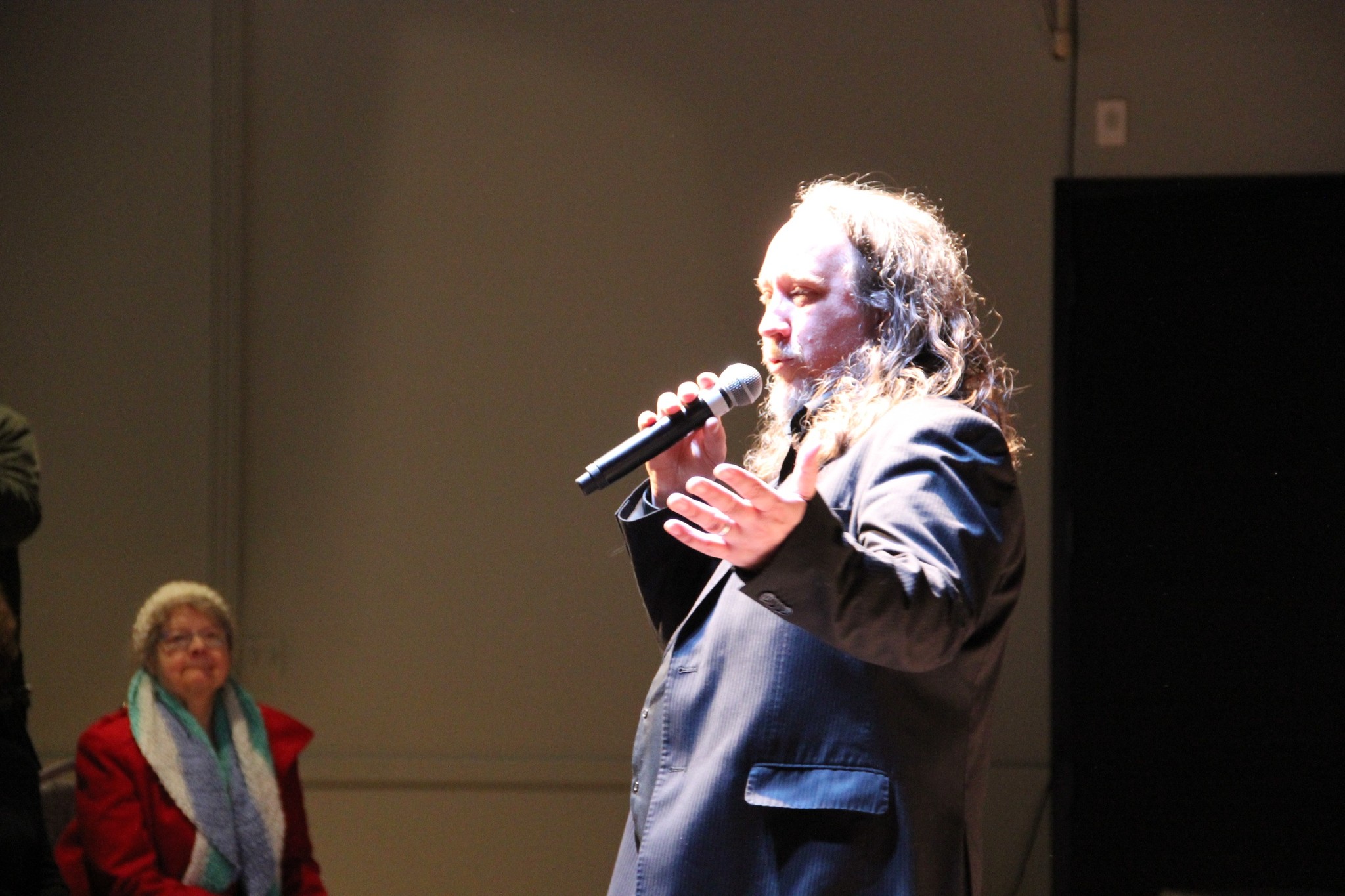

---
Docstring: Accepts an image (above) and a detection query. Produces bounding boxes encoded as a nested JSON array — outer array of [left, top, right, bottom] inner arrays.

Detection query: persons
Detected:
[[53, 581, 329, 896], [607, 179, 1028, 896], [0, 406, 72, 896]]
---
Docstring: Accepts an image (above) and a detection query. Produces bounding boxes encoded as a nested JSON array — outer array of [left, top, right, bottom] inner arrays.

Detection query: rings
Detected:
[[719, 517, 731, 537]]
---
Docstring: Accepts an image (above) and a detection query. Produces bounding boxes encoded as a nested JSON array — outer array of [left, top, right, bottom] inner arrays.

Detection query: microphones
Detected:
[[574, 362, 763, 495]]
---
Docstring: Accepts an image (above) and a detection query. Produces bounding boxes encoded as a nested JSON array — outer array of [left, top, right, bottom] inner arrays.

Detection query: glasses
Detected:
[[159, 631, 224, 651]]
